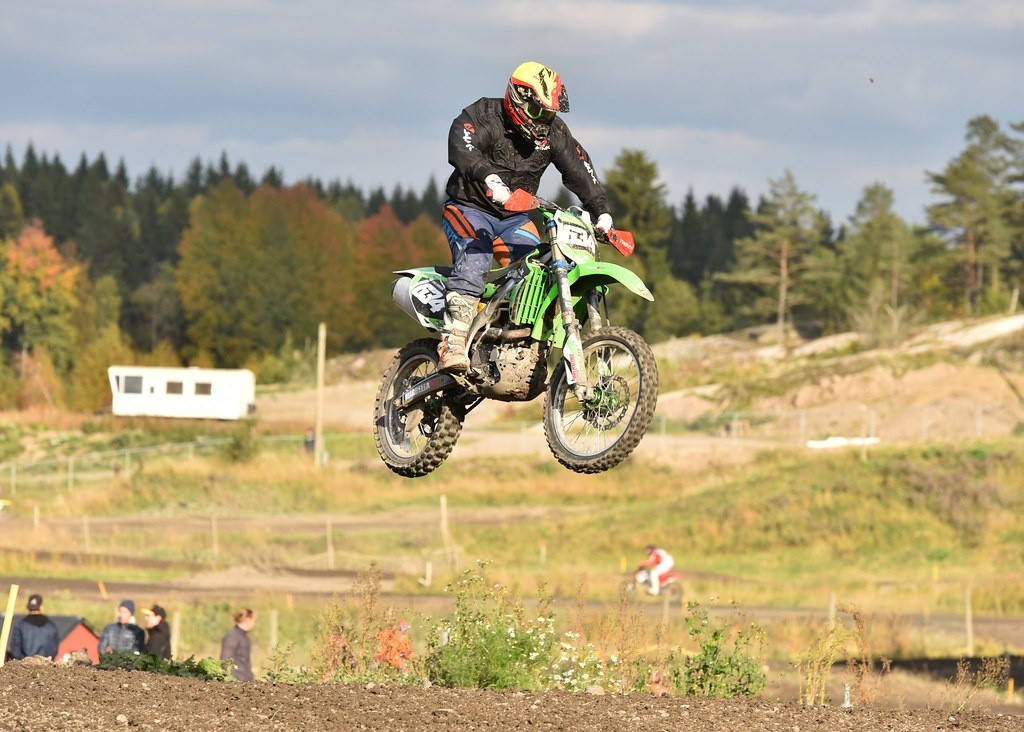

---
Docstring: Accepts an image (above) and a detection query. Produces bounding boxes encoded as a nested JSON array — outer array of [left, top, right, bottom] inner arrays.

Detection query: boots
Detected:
[[436, 291, 481, 371]]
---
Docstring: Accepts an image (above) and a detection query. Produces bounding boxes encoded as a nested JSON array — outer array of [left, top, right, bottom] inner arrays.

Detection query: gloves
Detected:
[[485, 174, 510, 205], [596, 213, 613, 233]]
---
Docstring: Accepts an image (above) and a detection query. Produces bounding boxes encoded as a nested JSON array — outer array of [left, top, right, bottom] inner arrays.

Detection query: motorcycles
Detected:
[[372, 188, 660, 477]]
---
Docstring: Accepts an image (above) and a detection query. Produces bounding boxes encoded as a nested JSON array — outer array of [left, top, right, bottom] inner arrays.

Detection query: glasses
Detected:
[[524, 102, 556, 119]]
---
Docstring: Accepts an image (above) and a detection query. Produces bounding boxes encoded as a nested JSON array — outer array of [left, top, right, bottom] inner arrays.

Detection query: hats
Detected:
[[29, 595, 41, 606], [120, 600, 134, 615], [142, 605, 166, 617]]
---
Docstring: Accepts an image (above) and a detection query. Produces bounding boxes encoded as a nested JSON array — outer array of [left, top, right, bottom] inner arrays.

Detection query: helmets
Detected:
[[503, 62, 570, 147]]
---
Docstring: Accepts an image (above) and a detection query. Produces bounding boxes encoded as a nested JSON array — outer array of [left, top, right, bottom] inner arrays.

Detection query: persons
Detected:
[[639, 545, 676, 596], [139, 604, 173, 662], [9, 591, 60, 664], [222, 608, 257, 681], [96, 598, 146, 662], [436, 60, 613, 374]]
